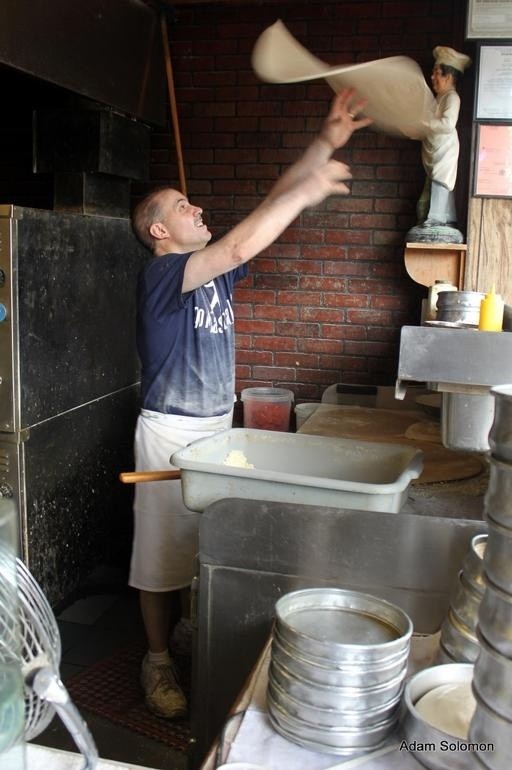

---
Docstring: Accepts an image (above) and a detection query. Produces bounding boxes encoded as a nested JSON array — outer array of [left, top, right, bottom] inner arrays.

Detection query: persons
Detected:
[[417, 43, 471, 227], [131, 87, 375, 721]]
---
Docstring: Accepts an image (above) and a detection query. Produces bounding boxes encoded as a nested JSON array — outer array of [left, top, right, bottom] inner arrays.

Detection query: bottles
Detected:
[[479, 282, 504, 332]]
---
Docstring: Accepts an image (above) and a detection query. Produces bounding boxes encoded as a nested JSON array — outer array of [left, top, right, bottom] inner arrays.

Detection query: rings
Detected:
[[346, 112, 356, 119]]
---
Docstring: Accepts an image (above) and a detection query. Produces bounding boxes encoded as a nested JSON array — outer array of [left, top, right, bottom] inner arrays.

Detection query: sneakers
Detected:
[[140, 654, 189, 720], [170, 618, 195, 656]]
